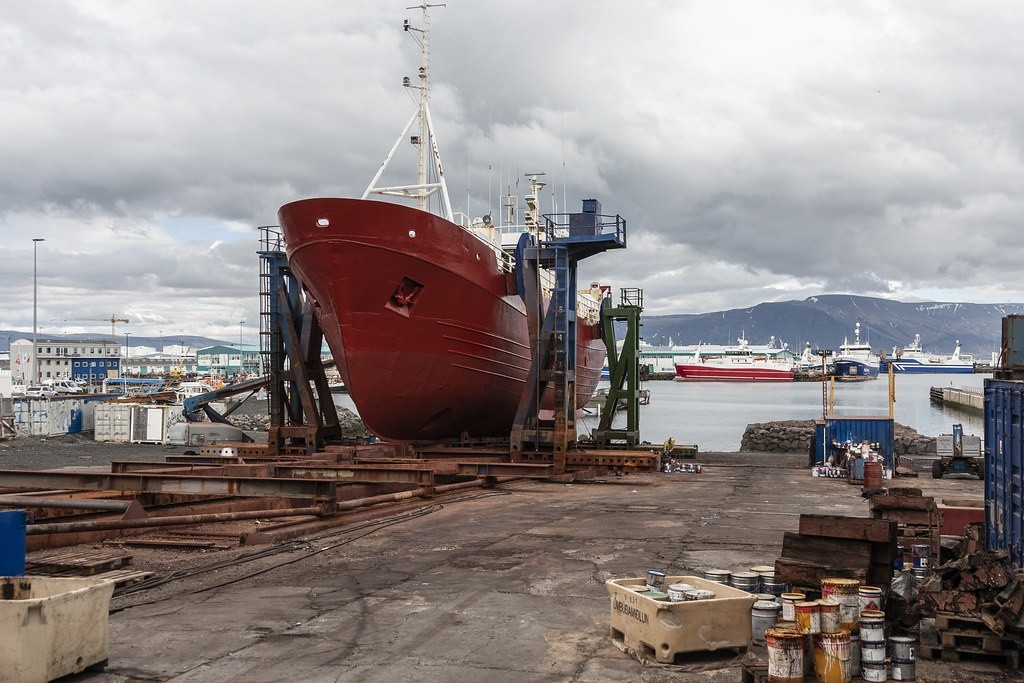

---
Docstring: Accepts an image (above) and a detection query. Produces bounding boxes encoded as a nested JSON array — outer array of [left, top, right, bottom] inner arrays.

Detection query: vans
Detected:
[[75, 379, 87, 387], [54, 380, 84, 395]]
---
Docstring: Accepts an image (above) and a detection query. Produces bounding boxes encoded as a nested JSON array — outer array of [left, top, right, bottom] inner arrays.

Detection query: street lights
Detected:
[[31, 237, 47, 386]]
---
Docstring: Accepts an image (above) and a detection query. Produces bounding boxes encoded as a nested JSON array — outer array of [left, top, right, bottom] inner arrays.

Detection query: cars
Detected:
[[26, 386, 58, 399]]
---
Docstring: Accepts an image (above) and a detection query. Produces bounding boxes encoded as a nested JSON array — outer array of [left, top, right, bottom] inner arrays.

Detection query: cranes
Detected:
[[50, 312, 128, 342]]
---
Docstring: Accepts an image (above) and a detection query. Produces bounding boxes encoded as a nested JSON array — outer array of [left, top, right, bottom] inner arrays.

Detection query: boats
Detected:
[[99, 366, 348, 407], [599, 321, 1004, 382], [278, 1, 631, 441]]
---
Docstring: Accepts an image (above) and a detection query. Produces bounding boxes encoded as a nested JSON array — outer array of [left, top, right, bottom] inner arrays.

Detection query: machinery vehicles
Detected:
[[931, 424, 985, 482]]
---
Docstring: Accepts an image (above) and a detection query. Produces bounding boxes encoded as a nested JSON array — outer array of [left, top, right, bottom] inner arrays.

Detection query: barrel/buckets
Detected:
[[863, 461, 883, 490], [629, 570, 714, 604], [851, 455, 871, 483], [704, 560, 916, 683]]
